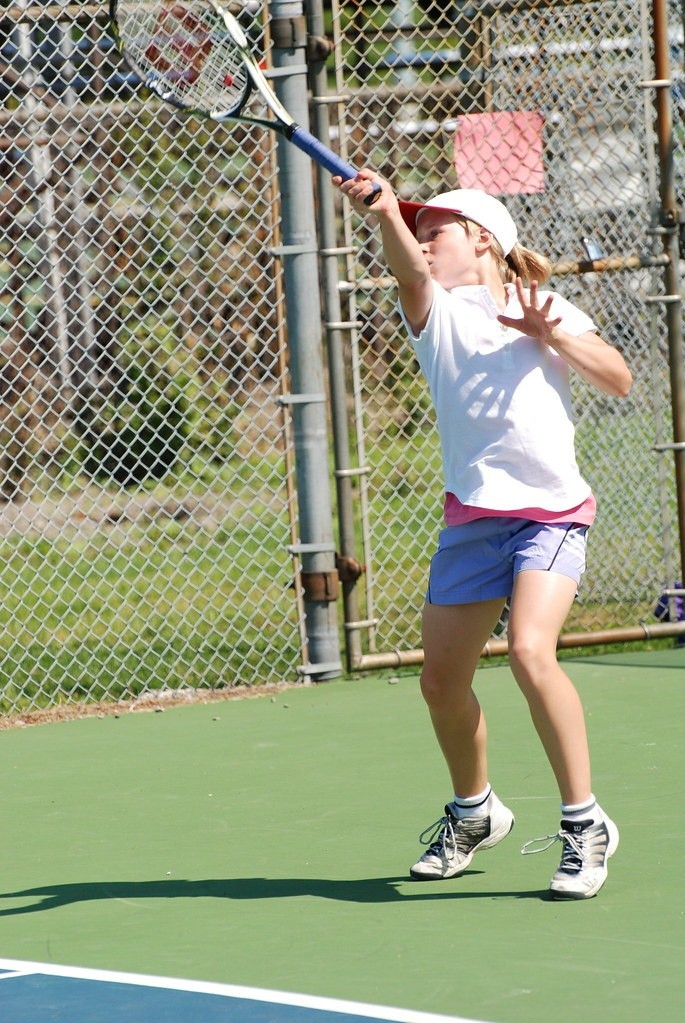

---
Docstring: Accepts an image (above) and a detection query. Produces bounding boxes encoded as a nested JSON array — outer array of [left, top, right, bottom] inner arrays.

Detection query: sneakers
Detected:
[[521, 802, 620, 899], [410, 792, 515, 879]]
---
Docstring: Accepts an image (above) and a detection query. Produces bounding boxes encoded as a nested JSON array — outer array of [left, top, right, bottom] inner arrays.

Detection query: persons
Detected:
[[333, 167, 632, 899]]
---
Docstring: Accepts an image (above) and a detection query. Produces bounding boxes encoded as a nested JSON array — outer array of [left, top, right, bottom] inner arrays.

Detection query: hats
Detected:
[[398, 188, 519, 260]]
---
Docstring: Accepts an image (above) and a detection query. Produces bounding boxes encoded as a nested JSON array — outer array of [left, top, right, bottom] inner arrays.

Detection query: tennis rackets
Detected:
[[106, 0, 384, 203]]
